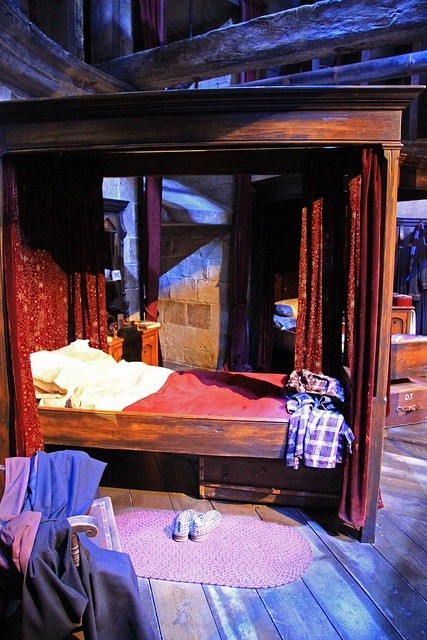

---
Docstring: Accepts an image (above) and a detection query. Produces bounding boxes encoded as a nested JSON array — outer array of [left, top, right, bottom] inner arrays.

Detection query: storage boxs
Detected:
[[384, 382, 427, 428]]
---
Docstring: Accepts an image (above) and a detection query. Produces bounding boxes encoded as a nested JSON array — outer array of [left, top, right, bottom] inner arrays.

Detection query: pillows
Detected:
[[25, 338, 116, 400]]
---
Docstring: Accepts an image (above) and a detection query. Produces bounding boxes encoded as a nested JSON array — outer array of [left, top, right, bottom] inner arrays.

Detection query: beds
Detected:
[[0, 102, 404, 546]]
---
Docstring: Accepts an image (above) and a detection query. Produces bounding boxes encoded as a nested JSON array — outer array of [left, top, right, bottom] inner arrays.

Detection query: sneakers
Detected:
[[189, 510, 222, 542], [173, 508, 197, 542]]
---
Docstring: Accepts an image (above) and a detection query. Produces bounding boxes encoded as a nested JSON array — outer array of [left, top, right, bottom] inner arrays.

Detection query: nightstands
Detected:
[[105, 320, 160, 369]]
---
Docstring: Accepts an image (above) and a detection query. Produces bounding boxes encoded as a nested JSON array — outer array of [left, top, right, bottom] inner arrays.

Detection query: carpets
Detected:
[[109, 509, 311, 588], [80, 495, 123, 554]]
